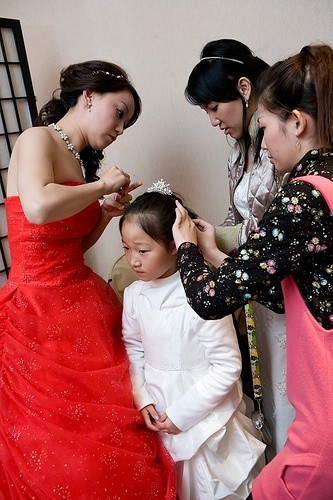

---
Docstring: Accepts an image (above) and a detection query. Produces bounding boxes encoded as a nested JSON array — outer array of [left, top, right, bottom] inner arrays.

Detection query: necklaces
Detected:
[[48, 122, 85, 179]]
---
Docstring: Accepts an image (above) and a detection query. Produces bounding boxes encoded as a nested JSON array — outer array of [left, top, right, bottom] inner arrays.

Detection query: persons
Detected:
[[185, 39, 291, 470], [173, 41, 333, 500], [1, 61, 185, 498], [116, 180, 268, 500]]
